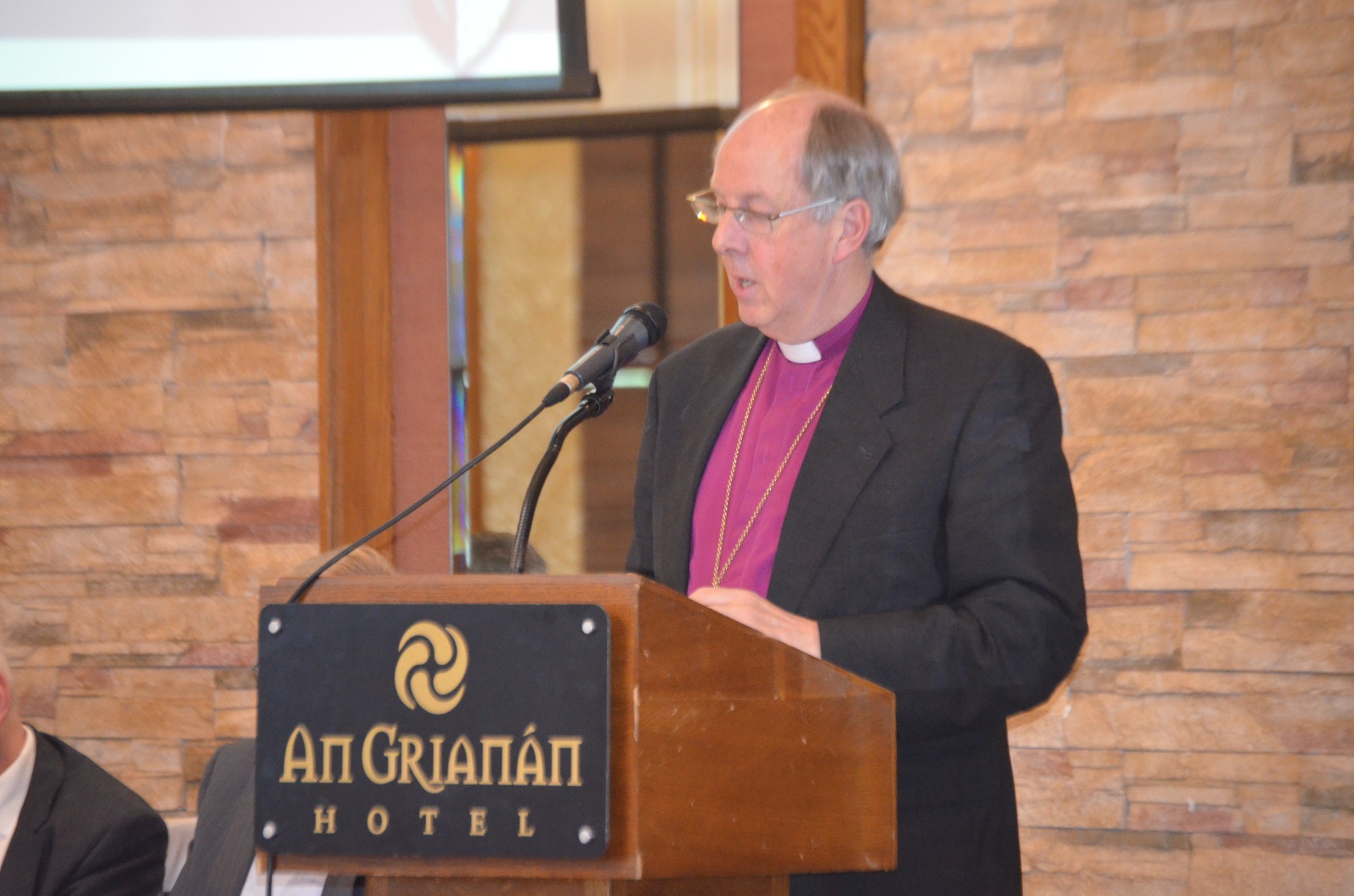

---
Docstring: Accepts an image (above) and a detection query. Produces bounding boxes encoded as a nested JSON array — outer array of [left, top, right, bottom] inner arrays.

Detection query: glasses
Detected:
[[686, 189, 844, 237]]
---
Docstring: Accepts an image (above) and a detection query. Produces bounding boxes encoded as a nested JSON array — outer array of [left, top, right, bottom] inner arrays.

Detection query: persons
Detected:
[[0, 651, 168, 896], [625, 87, 1089, 896], [168, 543, 399, 896]]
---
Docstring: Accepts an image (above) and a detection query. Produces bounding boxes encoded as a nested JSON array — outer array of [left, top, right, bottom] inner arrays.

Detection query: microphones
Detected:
[[542, 300, 668, 408]]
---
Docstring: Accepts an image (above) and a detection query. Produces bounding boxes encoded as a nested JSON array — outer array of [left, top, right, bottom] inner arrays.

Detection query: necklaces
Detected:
[[709, 343, 834, 586]]
